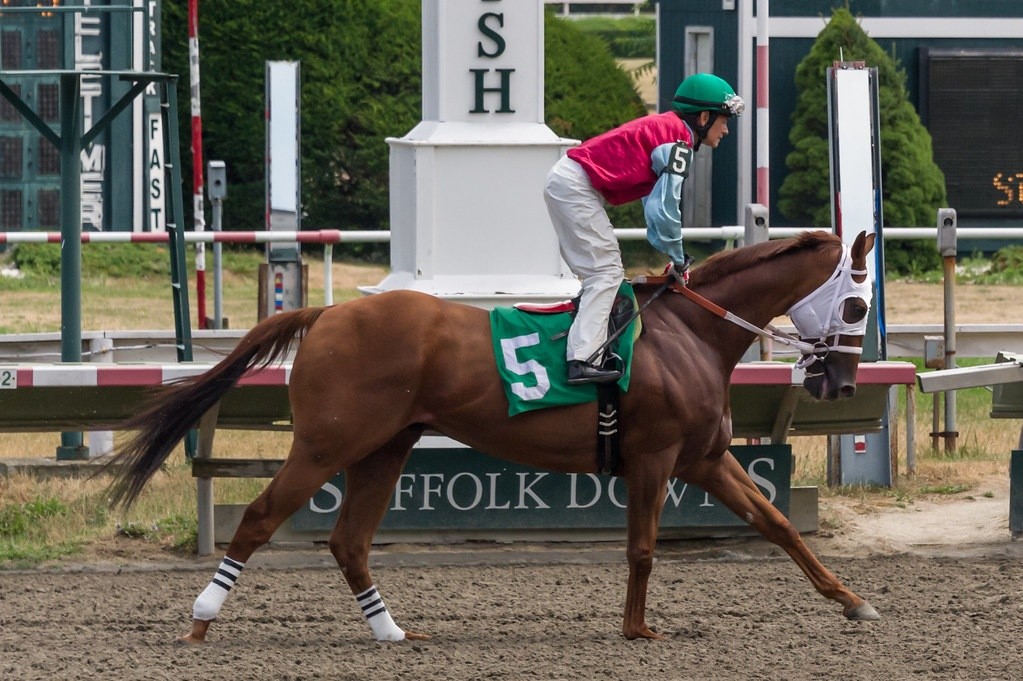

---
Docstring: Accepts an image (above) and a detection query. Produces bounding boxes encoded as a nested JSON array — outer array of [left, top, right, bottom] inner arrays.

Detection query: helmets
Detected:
[[671, 73, 745, 118]]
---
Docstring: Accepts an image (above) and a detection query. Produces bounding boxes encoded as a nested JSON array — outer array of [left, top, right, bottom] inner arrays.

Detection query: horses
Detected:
[[80, 230, 879, 648]]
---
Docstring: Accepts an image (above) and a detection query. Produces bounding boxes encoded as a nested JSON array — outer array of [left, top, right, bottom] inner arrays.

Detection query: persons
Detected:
[[542, 74, 744, 384]]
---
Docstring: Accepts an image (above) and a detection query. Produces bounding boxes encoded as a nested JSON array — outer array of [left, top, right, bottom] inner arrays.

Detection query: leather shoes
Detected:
[[567, 362, 619, 385]]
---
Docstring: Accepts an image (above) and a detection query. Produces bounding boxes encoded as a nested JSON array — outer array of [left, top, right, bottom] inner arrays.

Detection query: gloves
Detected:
[[661, 260, 689, 292]]
